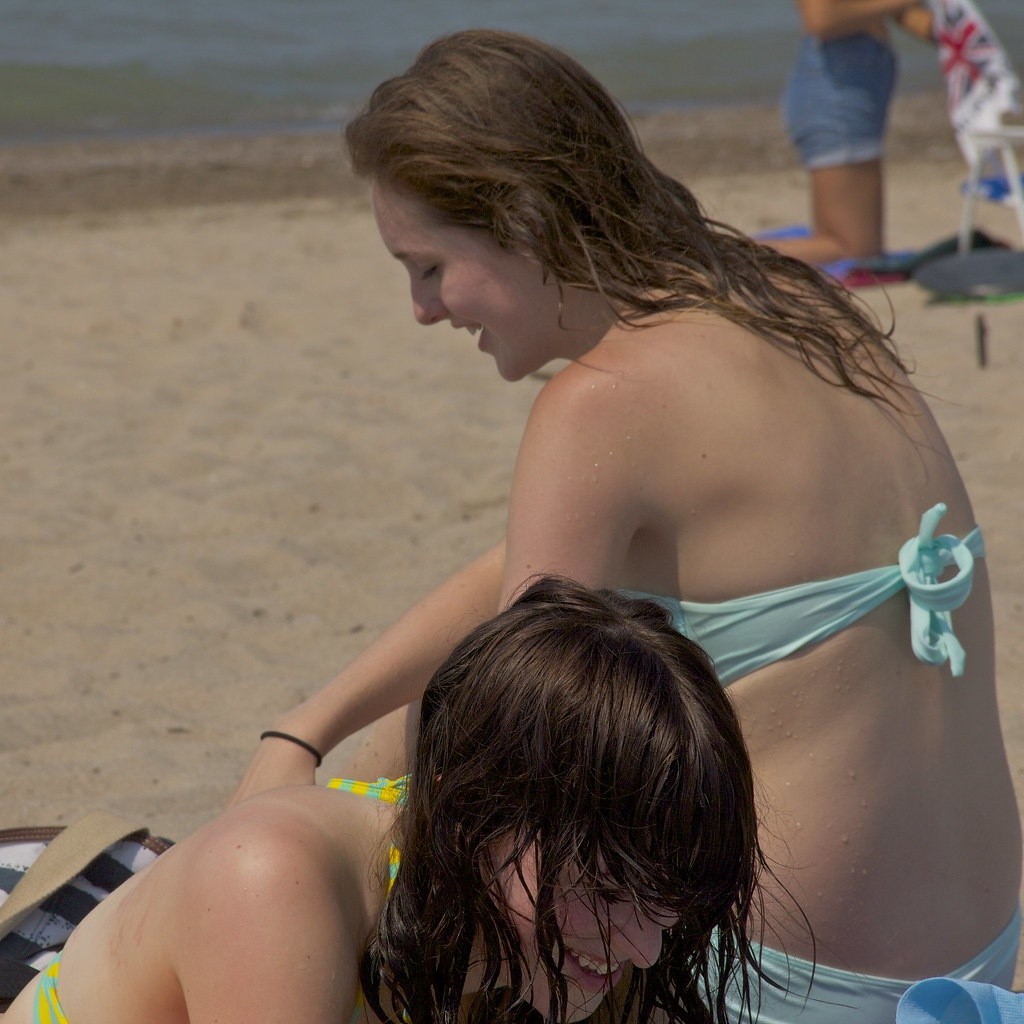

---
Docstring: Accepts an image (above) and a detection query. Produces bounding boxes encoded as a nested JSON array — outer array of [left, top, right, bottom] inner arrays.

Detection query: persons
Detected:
[[0, 576, 818, 1024], [230, 29, 1024, 1024], [763, 0, 935, 263]]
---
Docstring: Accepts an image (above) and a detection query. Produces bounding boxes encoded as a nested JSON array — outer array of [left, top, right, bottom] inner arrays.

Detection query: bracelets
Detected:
[[260, 732, 322, 768]]
